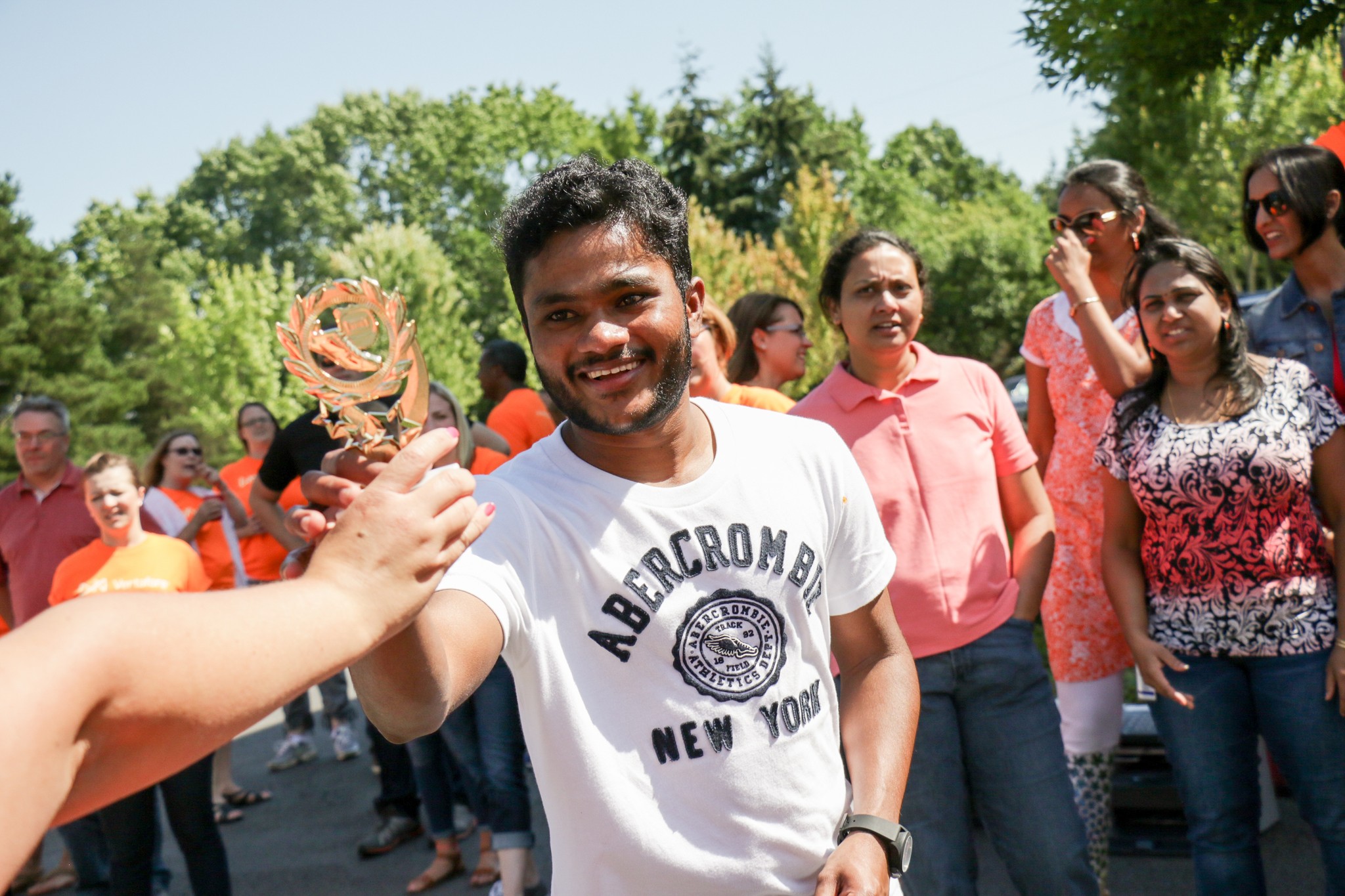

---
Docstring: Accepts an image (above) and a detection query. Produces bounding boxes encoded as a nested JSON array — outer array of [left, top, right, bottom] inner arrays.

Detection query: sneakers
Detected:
[[266, 737, 315, 772], [329, 728, 359, 761], [449, 805, 476, 841], [357, 815, 422, 859]]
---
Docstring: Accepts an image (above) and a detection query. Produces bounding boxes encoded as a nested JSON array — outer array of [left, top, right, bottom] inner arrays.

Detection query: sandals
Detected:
[[223, 786, 271, 803], [467, 845, 502, 888], [213, 801, 244, 823], [404, 853, 464, 895]]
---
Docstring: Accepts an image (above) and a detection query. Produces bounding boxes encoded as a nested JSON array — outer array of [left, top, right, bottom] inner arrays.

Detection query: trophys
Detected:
[[275, 276, 431, 452]]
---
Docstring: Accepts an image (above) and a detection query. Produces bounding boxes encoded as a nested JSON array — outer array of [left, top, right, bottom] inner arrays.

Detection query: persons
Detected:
[[287, 158, 923, 896], [1018, 160, 1183, 896], [1089, 239, 1345, 896], [0, 340, 560, 896], [790, 234, 1094, 896], [680, 292, 815, 414], [1240, 141, 1345, 545]]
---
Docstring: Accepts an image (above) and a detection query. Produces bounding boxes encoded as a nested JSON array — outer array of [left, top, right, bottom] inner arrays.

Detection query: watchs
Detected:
[[837, 813, 912, 879]]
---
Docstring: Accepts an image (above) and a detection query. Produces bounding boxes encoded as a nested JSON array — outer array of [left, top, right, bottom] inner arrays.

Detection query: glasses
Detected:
[[763, 322, 805, 336], [1049, 208, 1121, 240], [165, 446, 203, 457], [241, 415, 273, 430], [16, 431, 63, 441], [1243, 189, 1292, 223]]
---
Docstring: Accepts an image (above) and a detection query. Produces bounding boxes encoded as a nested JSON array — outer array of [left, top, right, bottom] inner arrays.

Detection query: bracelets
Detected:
[[1335, 638, 1345, 648], [1068, 296, 1103, 323]]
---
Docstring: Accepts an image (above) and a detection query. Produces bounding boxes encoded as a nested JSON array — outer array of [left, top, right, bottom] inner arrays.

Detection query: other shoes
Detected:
[[488, 873, 546, 896]]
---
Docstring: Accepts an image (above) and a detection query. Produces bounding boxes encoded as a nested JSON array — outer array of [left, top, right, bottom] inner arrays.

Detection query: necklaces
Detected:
[[1166, 379, 1230, 425]]
